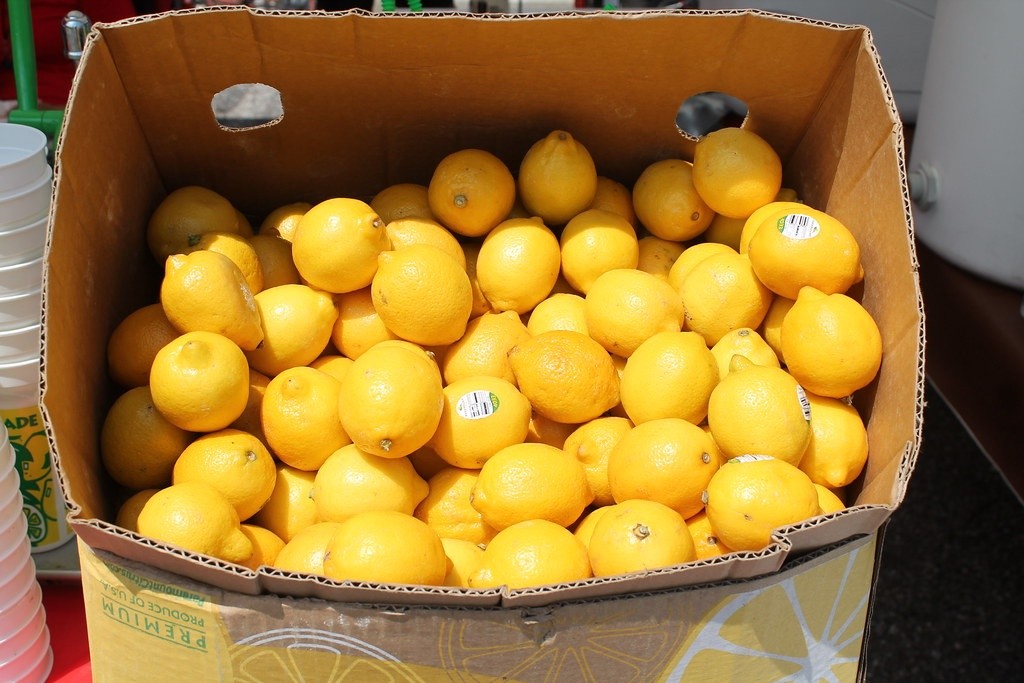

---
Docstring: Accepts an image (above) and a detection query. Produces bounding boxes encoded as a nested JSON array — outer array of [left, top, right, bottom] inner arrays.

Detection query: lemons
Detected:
[[100, 126, 883, 587]]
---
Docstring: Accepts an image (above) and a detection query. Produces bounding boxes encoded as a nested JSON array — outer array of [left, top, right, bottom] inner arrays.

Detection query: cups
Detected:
[[0, 125, 109, 683]]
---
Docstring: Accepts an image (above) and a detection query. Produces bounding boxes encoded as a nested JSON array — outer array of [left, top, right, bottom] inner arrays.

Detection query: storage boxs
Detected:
[[36, 4, 926, 683]]
